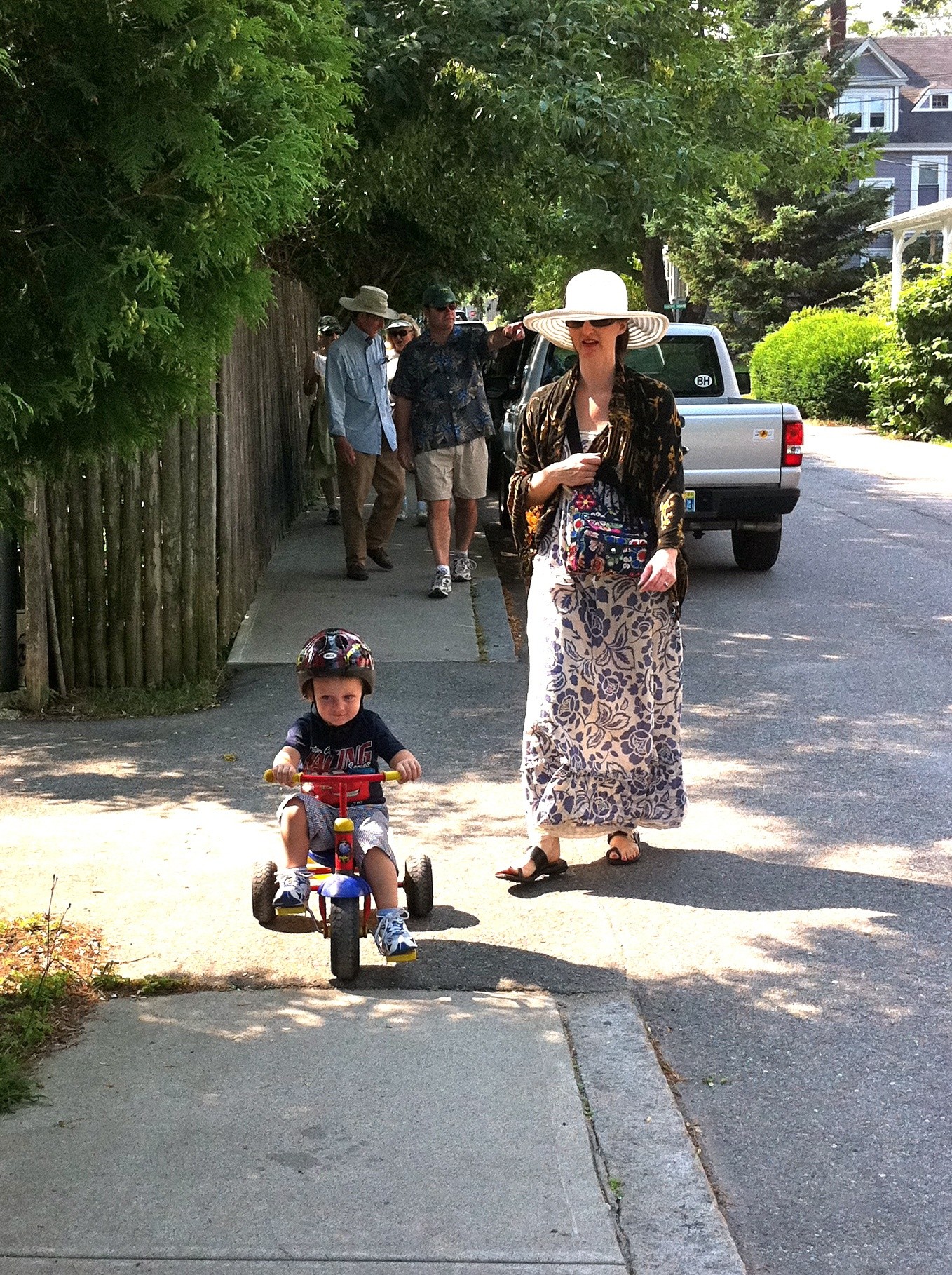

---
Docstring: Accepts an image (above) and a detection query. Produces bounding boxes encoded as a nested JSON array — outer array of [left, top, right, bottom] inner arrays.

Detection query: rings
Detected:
[[664, 581, 669, 587]]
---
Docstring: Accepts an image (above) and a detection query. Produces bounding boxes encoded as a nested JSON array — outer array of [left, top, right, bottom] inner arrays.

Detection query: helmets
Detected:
[[296, 628, 374, 695]]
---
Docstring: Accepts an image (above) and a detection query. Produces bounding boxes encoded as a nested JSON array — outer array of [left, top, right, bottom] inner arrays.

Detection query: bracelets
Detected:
[[506, 335, 512, 340]]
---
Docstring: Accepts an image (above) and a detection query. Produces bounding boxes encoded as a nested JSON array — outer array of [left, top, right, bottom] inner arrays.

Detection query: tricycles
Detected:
[[249, 769, 435, 982]]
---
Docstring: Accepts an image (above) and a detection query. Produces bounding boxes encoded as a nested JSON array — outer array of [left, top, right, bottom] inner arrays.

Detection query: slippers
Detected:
[[606, 830, 643, 866], [495, 846, 568, 884]]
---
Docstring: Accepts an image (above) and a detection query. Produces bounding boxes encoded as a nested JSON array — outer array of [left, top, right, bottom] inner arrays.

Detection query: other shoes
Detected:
[[366, 547, 393, 569], [399, 505, 409, 521], [349, 564, 368, 580], [327, 509, 341, 525], [417, 509, 428, 527]]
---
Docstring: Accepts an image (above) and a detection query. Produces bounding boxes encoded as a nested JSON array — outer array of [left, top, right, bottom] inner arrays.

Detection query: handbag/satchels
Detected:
[[565, 511, 650, 579]]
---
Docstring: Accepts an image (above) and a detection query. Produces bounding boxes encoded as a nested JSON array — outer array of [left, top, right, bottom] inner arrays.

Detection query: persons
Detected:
[[303, 315, 343, 527], [272, 629, 421, 962], [325, 285, 404, 580], [382, 312, 430, 524], [390, 284, 529, 601], [495, 306, 688, 884]]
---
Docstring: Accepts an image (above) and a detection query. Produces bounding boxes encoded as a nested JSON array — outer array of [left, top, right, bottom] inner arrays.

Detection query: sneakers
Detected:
[[451, 553, 474, 582], [430, 568, 452, 598], [273, 868, 310, 906], [377, 912, 419, 963]]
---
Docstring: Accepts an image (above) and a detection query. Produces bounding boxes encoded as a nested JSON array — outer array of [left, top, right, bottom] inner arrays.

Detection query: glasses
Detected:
[[390, 330, 413, 337], [428, 305, 458, 311], [324, 330, 342, 336], [568, 319, 618, 326]]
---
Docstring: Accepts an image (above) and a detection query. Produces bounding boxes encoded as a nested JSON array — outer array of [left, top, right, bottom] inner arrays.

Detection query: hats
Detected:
[[422, 283, 460, 307], [386, 320, 413, 329], [339, 286, 399, 320], [317, 315, 340, 332], [523, 269, 670, 351]]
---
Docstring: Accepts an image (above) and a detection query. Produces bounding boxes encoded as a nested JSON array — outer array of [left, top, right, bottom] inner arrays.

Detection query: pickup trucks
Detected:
[[501, 322, 803, 573]]
